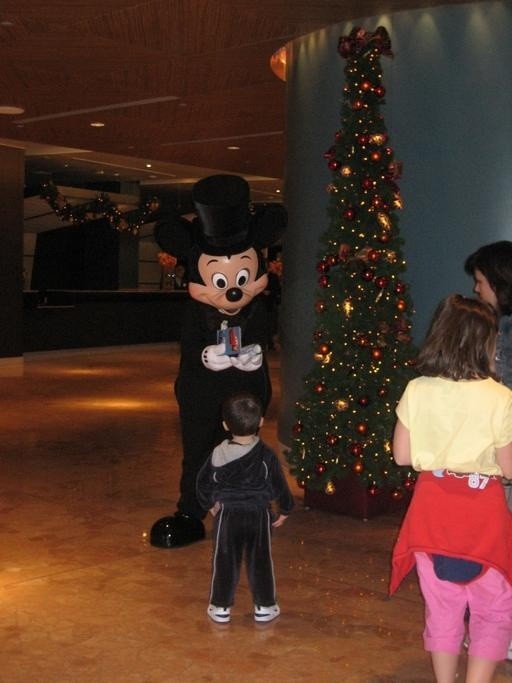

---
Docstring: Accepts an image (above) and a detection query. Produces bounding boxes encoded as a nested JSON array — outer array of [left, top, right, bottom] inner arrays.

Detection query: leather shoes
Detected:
[[150, 511, 206, 548]]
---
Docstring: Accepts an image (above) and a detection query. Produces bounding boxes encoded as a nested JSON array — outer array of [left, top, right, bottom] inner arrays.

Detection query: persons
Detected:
[[391, 293, 512, 682], [464, 241, 511, 389], [195, 393, 295, 623]]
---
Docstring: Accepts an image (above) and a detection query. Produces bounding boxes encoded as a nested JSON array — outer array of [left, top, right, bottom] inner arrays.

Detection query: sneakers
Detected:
[[207, 604, 231, 623], [254, 604, 281, 622]]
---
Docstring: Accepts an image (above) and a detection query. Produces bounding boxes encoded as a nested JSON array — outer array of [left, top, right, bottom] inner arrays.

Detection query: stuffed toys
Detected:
[[150, 176, 294, 548]]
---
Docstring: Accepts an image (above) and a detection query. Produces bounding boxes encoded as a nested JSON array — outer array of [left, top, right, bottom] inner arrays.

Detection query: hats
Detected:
[[154, 175, 289, 257]]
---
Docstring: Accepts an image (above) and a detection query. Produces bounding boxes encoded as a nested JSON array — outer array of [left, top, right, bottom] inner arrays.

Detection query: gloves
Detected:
[[201, 343, 263, 372]]
